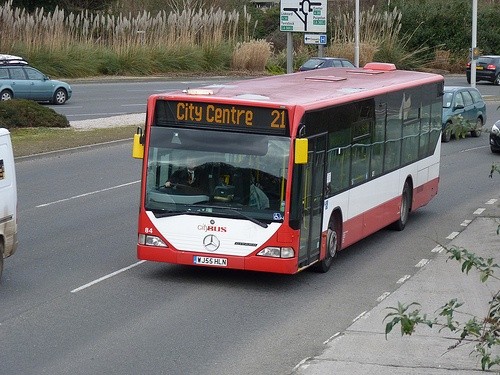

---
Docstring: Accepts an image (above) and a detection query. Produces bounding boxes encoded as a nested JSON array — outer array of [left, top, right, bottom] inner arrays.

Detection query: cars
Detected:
[[466, 55, 500, 86], [0, 54, 72, 105], [299, 57, 356, 72]]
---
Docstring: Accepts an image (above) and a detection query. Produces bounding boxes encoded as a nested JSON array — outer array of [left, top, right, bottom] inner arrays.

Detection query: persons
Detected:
[[164, 155, 210, 196]]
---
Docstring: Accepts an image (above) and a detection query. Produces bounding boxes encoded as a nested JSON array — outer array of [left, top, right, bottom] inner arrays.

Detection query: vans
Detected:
[[442, 86, 487, 143]]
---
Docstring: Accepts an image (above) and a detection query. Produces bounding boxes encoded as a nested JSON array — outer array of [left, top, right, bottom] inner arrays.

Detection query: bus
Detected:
[[132, 63, 445, 275]]
